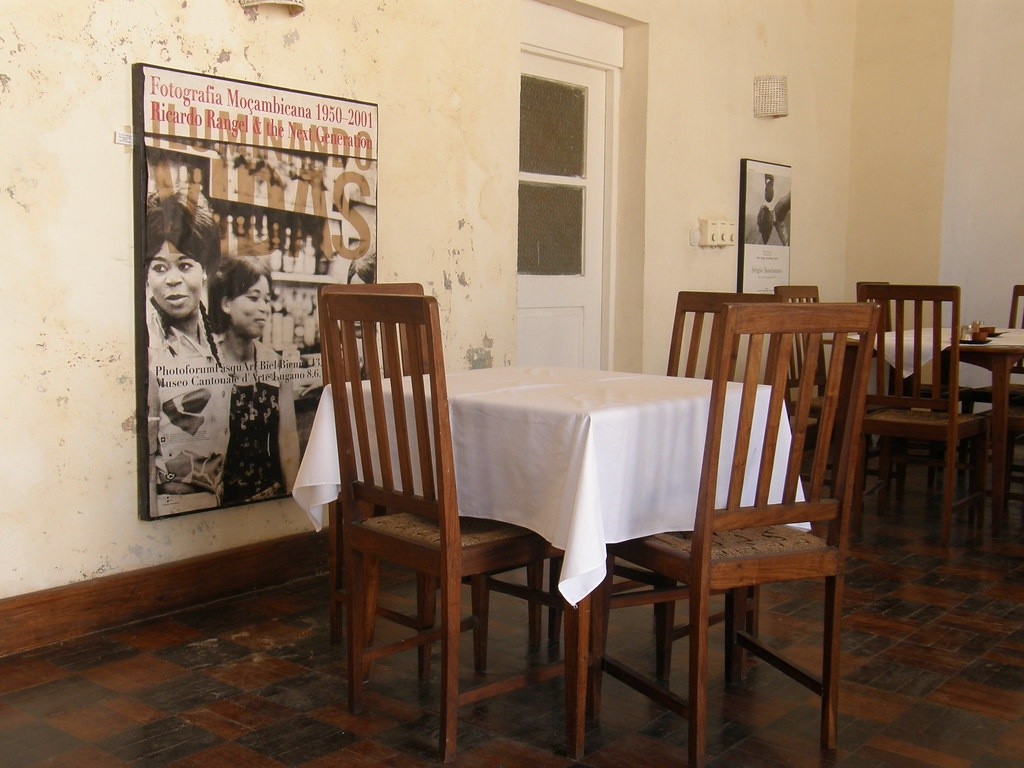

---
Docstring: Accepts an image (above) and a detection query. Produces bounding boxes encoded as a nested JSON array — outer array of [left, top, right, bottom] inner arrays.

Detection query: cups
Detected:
[[979, 326, 995, 335], [960, 323, 972, 341], [972, 332, 988, 342], [971, 321, 984, 338]]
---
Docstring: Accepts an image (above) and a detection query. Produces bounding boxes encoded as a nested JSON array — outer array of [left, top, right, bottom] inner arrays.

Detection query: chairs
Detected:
[[316, 281, 1024, 768]]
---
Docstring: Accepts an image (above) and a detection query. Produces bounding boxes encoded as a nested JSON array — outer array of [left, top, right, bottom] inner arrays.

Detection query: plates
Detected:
[[950, 338, 992, 344], [987, 333, 1002, 337]]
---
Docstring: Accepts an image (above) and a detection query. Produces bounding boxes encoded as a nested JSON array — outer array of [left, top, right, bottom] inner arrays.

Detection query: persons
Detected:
[[302, 253, 376, 397], [145, 193, 301, 516]]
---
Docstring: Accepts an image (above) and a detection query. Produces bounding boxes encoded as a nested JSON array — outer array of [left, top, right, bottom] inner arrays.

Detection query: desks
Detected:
[[821, 327, 1024, 541], [290, 368, 811, 760]]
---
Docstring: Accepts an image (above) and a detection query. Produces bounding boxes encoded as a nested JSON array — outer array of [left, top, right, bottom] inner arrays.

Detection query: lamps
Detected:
[[240, 0, 305, 17], [753, 76, 788, 118]]
